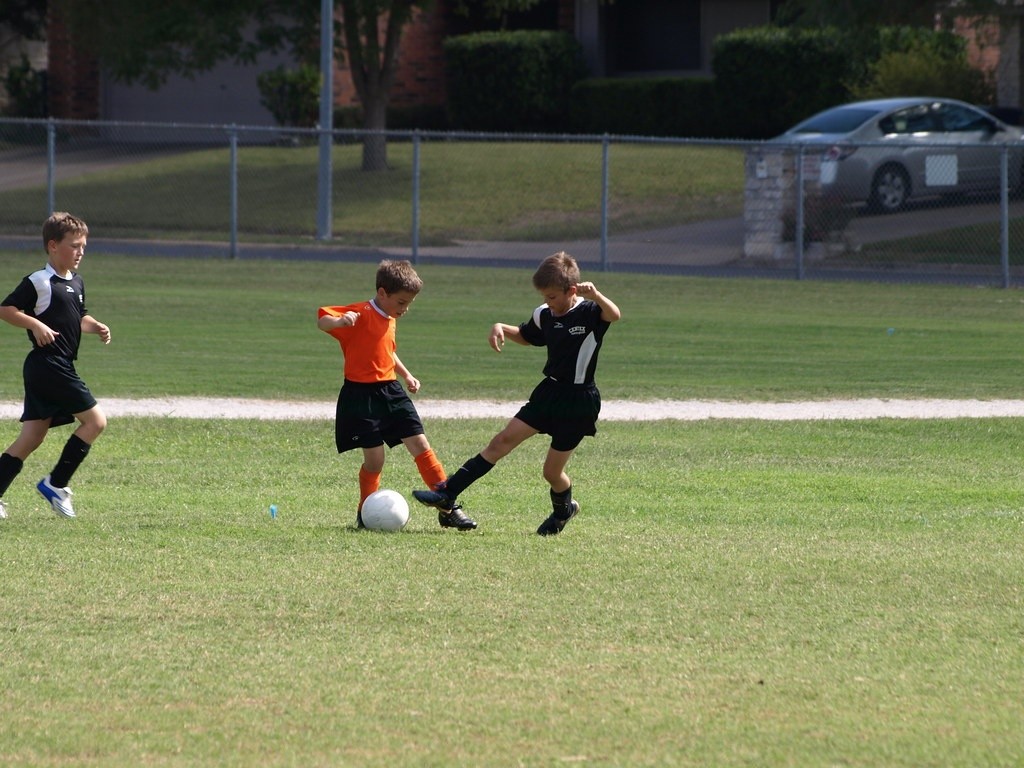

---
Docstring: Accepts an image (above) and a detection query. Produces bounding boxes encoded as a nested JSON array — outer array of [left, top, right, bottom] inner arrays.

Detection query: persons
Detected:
[[0, 212, 111, 519], [413, 252, 620, 536], [317, 260, 477, 531]]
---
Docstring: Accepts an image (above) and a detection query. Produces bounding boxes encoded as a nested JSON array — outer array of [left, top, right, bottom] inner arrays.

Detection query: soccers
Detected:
[[362, 488, 410, 534]]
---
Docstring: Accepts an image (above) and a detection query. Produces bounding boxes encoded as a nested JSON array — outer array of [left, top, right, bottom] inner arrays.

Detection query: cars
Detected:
[[743, 97, 1024, 218]]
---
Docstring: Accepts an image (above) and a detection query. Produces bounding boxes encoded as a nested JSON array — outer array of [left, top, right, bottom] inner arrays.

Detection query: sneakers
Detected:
[[357, 511, 365, 528], [36, 472, 76, 519], [412, 481, 457, 515], [0, 499, 8, 519], [439, 501, 477, 531], [537, 500, 580, 537]]
